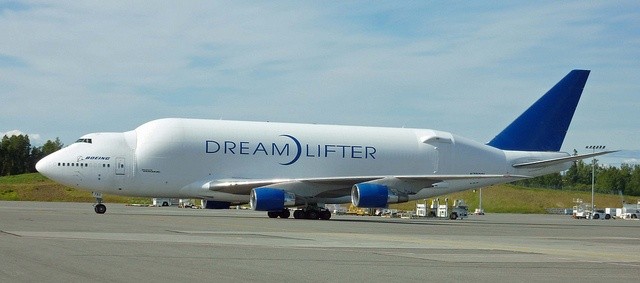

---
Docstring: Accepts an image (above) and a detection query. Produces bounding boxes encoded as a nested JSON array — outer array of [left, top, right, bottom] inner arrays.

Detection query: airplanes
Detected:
[[36, 69, 622, 219]]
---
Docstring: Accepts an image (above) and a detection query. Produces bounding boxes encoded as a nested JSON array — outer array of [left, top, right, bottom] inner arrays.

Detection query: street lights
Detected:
[[585, 145, 605, 208]]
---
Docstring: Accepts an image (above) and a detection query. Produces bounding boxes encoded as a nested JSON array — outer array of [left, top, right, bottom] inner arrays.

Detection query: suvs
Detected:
[[592, 208, 610, 219]]
